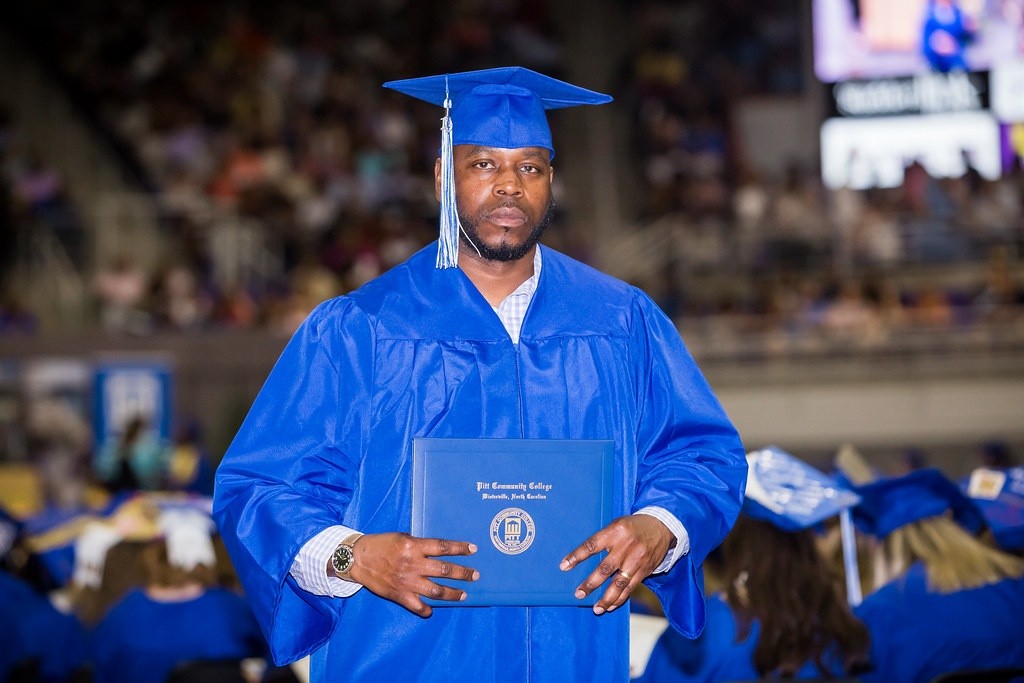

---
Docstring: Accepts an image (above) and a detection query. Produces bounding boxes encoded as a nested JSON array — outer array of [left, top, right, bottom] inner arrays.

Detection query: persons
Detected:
[[1, 415, 311, 683], [210, 68, 752, 682], [634, 438, 1024, 683], [5, 0, 575, 333], [617, 1, 1024, 361]]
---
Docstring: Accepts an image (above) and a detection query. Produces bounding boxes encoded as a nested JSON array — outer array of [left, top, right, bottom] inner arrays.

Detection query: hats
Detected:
[[383, 68, 616, 161], [0, 443, 1021, 606]]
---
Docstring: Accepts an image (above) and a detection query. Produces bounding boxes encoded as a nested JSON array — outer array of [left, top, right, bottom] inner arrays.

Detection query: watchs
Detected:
[[331, 532, 363, 583]]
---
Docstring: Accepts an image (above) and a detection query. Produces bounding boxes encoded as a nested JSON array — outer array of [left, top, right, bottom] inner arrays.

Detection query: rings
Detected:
[[618, 569, 632, 584]]
